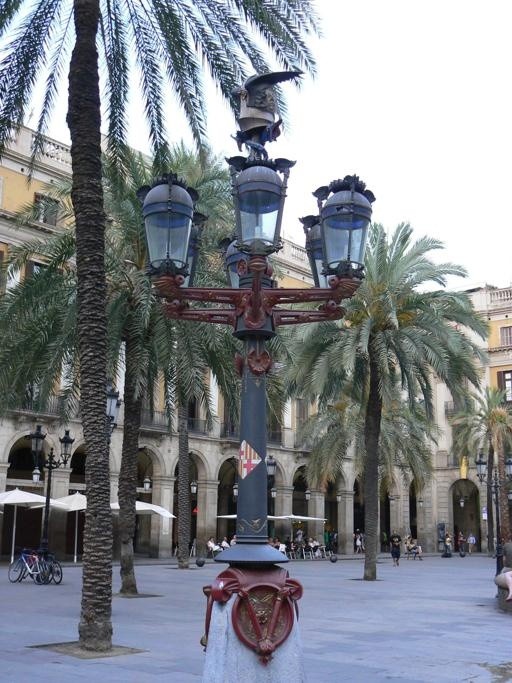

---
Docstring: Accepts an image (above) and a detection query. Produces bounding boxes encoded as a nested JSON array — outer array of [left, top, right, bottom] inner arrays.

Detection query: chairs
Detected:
[[276, 543, 326, 560], [206, 544, 223, 560]]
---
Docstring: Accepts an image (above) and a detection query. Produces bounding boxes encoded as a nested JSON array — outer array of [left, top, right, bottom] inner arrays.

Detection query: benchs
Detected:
[[405, 552, 420, 559]]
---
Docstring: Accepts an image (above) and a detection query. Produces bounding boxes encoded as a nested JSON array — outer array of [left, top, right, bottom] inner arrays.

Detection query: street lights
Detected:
[[473, 444, 512, 574], [27, 421, 76, 550], [137, 57, 380, 682]]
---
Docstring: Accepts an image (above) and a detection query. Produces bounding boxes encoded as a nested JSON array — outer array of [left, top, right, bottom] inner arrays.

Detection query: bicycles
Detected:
[[6, 545, 65, 585]]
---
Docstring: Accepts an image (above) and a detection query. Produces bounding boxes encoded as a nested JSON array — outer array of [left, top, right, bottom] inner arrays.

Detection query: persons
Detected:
[[190, 538, 197, 557], [441, 529, 479, 557], [381, 530, 423, 567], [267, 528, 338, 560], [492, 536, 512, 601], [354, 528, 366, 554], [206, 534, 236, 554], [135, 499, 177, 519]]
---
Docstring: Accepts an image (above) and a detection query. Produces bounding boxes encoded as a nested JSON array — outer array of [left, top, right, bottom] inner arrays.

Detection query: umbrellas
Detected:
[[0, 487, 47, 565], [268, 515, 288, 524], [277, 512, 327, 543], [26, 491, 87, 563], [215, 515, 237, 519], [110, 503, 120, 513]]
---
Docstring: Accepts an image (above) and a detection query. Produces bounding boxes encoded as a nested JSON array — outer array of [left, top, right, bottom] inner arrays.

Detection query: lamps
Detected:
[[459, 493, 465, 508], [335, 489, 342, 502], [388, 493, 397, 505], [142, 454, 152, 491], [418, 490, 426, 508], [190, 459, 198, 494], [232, 468, 239, 496], [270, 474, 277, 498], [31, 466, 40, 485], [304, 487, 311, 501]]
